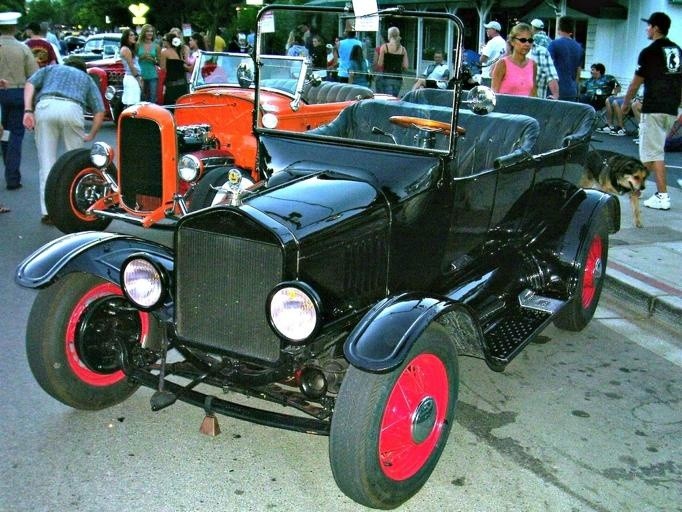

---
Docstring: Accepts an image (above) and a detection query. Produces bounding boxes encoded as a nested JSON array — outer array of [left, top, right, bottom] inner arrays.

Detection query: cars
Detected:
[[81, 56, 226, 124], [12, 0, 626, 509], [65, 20, 163, 62], [44, 51, 397, 238]]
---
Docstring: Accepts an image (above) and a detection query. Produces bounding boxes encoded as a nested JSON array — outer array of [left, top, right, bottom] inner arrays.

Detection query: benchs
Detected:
[[259, 74, 375, 109], [400, 88, 597, 192], [305, 101, 540, 234]]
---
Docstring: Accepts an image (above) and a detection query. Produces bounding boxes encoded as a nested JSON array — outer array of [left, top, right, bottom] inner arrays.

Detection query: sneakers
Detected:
[[643, 192, 671, 210], [610, 125, 627, 137], [595, 124, 614, 134], [41, 215, 54, 225]]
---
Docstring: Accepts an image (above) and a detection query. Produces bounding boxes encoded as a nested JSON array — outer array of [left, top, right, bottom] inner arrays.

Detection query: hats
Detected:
[[531, 19, 544, 29], [0, 12, 22, 25], [641, 12, 671, 29], [483, 21, 502, 32]]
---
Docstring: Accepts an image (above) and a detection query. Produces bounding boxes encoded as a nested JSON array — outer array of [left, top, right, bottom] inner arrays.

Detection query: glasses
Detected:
[[514, 37, 534, 44]]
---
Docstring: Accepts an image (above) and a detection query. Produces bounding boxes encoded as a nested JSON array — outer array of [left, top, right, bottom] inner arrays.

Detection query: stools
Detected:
[[594, 106, 609, 129], [622, 114, 639, 132]]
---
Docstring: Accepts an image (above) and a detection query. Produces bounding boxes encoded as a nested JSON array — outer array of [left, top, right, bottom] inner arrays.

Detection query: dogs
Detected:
[[579, 149, 649, 228]]
